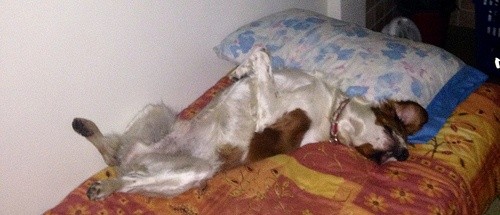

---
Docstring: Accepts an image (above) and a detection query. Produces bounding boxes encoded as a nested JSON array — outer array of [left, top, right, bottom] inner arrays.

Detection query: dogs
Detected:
[[72, 44, 429, 201]]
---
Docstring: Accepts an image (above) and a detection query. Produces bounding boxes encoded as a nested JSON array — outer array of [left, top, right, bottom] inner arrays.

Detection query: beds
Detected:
[[43, 64, 500, 215]]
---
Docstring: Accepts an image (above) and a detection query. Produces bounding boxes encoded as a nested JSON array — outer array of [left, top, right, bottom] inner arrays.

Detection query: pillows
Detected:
[[213, 7, 489, 145]]
